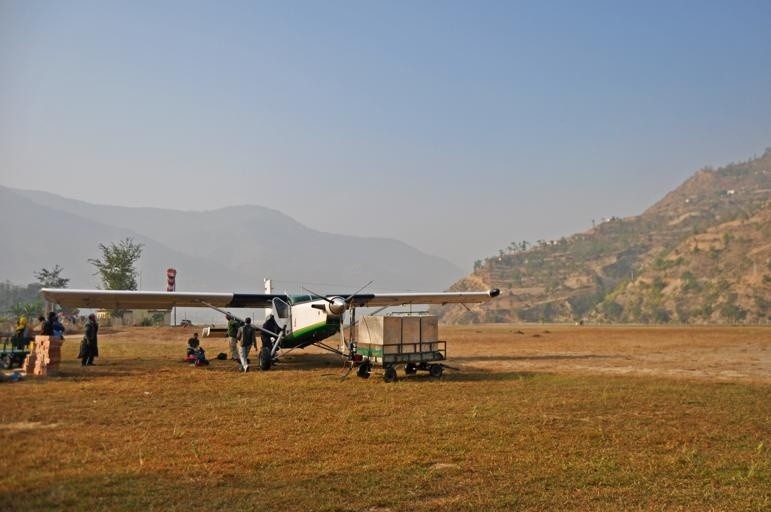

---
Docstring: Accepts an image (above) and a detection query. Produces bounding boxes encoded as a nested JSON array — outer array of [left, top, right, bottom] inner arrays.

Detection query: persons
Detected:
[[236, 317, 260, 374], [11, 311, 67, 369], [187, 332, 205, 358], [77, 313, 100, 367], [224, 311, 240, 361]]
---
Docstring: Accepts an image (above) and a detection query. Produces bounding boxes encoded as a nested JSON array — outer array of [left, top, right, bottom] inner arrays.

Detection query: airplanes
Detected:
[[40, 287, 499, 369]]
